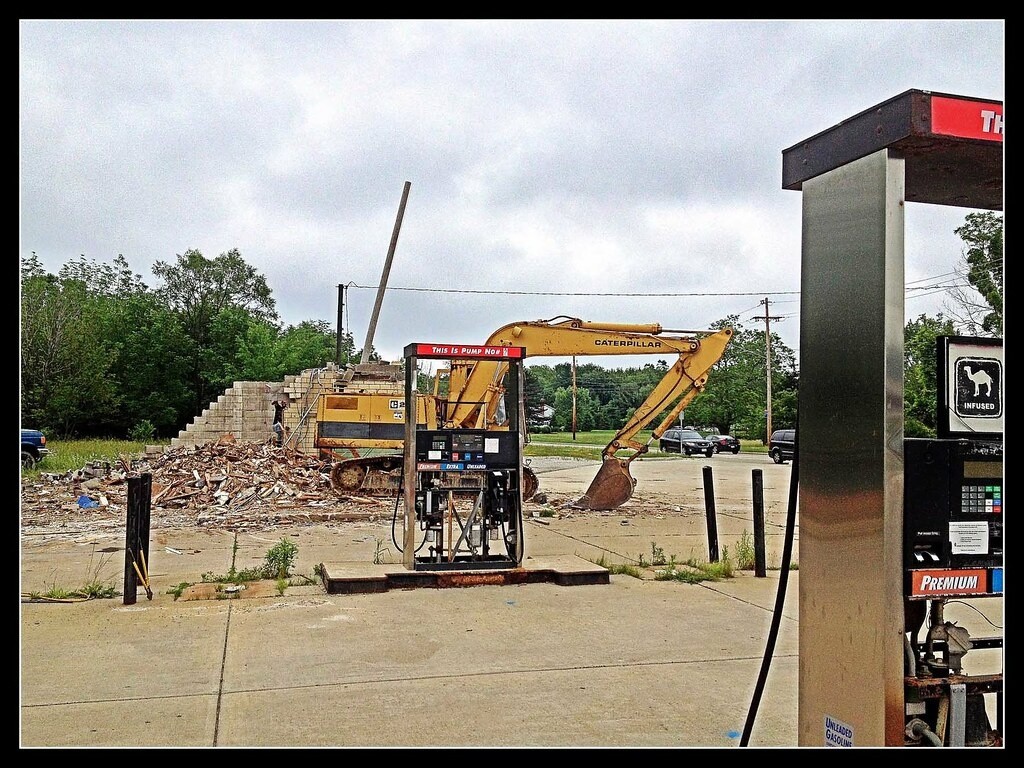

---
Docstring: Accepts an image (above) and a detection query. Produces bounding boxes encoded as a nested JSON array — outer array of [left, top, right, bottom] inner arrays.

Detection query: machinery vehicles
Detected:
[[313, 316, 733, 514]]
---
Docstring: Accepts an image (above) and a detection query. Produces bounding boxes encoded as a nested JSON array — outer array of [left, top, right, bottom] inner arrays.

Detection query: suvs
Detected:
[[659, 429, 715, 458], [768, 430, 796, 464]]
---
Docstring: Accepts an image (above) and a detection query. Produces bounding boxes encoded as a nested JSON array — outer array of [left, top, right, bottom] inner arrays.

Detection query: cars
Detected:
[[21, 429, 50, 472], [704, 435, 741, 455]]
